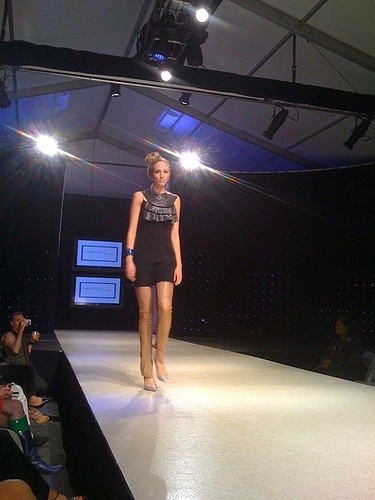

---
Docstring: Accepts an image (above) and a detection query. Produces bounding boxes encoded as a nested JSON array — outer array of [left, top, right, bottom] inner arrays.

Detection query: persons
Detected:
[[0, 358, 87, 500], [124, 151, 183, 391], [0, 310, 48, 395], [312, 316, 359, 382]]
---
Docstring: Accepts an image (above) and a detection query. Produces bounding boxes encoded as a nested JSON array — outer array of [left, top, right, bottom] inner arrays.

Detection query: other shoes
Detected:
[[144, 376, 157, 391], [154, 356, 167, 382], [30, 435, 50, 447]]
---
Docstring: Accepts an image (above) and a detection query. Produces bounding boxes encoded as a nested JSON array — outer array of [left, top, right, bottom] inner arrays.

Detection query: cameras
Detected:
[[26, 319, 31, 326]]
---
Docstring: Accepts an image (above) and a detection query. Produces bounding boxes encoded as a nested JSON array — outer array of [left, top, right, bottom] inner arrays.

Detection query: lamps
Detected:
[[179, 92, 192, 105], [262, 103, 300, 141], [0, 69, 12, 109], [343, 114, 374, 150], [109, 83, 121, 98]]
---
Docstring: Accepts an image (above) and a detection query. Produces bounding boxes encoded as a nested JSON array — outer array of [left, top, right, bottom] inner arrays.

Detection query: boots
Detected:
[[7, 414, 63, 475]]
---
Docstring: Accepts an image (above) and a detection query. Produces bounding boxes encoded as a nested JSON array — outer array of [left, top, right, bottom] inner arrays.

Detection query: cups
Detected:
[[31, 330, 39, 340]]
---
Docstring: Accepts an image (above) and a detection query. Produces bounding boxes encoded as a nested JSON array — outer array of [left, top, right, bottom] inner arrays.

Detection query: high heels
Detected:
[[27, 395, 52, 410], [27, 406, 49, 425]]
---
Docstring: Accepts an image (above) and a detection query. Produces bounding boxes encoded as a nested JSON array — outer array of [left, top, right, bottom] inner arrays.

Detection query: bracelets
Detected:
[[28, 341, 34, 344], [125, 249, 136, 257]]
